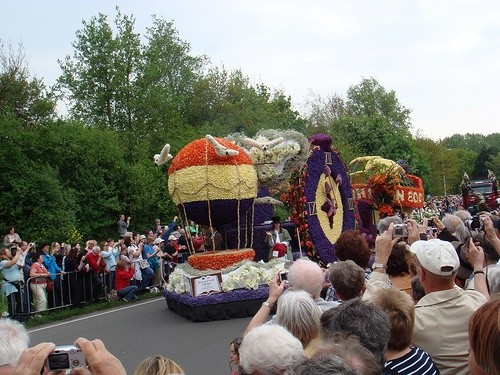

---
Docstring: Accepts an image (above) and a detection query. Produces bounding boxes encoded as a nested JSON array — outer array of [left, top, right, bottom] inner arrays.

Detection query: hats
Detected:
[[271, 216, 282, 224], [168, 235, 178, 241], [139, 235, 145, 240], [155, 238, 165, 244], [410, 239, 460, 276]]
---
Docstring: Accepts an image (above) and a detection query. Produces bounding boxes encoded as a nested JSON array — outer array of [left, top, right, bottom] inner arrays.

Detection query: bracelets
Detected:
[[474, 270, 484, 275], [372, 263, 387, 269]]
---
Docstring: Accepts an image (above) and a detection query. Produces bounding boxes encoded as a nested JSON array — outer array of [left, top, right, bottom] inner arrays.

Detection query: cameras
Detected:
[[465, 217, 485, 232], [278, 271, 289, 283], [392, 224, 409, 238], [43, 345, 87, 372], [431, 228, 437, 234]]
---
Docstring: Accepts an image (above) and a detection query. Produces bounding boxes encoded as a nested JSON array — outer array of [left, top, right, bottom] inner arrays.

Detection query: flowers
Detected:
[[279, 166, 316, 265], [367, 163, 410, 214]]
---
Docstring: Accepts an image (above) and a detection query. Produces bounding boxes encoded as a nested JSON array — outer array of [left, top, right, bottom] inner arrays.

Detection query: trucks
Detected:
[[464, 179, 500, 216]]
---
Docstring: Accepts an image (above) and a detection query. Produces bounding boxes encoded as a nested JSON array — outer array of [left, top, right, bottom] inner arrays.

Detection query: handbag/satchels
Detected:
[[46, 279, 52, 291], [12, 281, 23, 290]]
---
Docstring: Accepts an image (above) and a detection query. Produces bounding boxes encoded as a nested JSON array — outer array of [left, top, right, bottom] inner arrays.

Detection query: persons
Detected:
[[277, 290, 324, 342], [0, 319, 186, 375], [16, 216, 223, 317], [336, 229, 370, 278], [229, 337, 244, 375], [3, 226, 22, 249], [243, 259, 342, 335], [329, 259, 366, 300], [117, 213, 131, 238], [265, 216, 293, 261], [0, 247, 24, 320], [286, 298, 392, 375], [238, 324, 304, 375], [361, 191, 500, 375], [370, 287, 440, 375]]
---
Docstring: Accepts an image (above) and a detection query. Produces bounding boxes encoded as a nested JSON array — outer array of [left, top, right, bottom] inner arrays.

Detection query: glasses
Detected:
[[104, 245, 109, 247]]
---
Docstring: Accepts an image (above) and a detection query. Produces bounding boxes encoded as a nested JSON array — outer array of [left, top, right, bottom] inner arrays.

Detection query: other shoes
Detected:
[[145, 286, 151, 290], [120, 298, 127, 303]]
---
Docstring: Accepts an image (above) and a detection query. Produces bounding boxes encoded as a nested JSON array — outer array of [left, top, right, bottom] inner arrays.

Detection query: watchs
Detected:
[[263, 302, 272, 310]]
